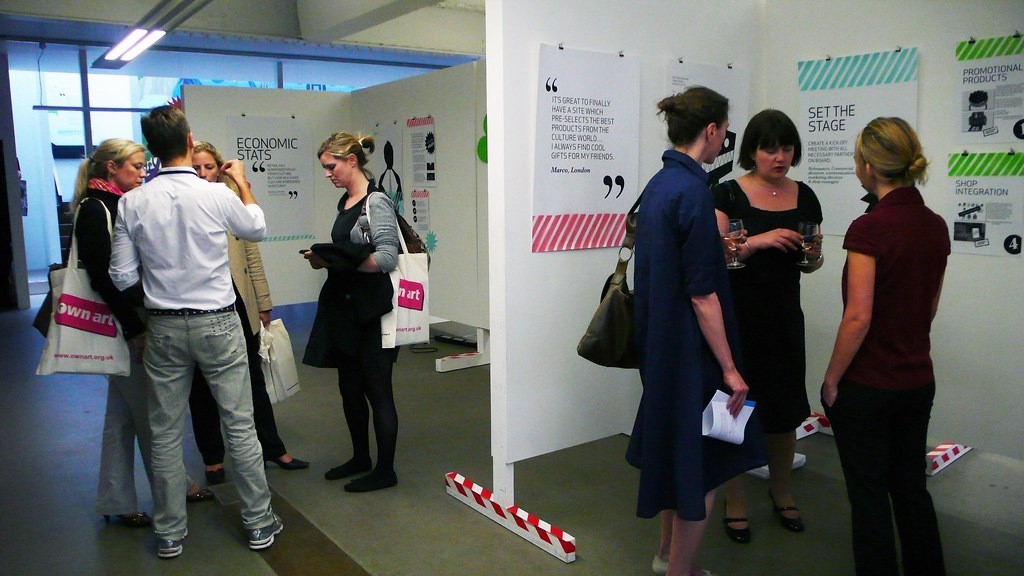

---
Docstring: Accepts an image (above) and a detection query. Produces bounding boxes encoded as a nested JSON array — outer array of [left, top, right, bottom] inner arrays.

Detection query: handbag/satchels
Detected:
[[366, 192, 430, 349], [35, 197, 130, 377], [258, 319, 301, 404], [576, 273, 643, 369]]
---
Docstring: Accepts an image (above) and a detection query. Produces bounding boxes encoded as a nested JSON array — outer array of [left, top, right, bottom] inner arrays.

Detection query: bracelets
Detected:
[[745, 242, 755, 254]]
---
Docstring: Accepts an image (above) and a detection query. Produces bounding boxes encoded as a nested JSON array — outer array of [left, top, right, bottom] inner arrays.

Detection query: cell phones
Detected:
[[299, 249, 310, 254]]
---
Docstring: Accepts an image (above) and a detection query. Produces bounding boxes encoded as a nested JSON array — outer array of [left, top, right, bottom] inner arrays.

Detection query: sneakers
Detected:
[[157, 527, 188, 557], [247, 513, 284, 549]]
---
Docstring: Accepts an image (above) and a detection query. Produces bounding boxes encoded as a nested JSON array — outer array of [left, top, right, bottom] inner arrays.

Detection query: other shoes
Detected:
[[722, 496, 752, 543], [651, 555, 712, 576], [344, 470, 399, 492], [324, 453, 373, 481], [770, 494, 805, 532]]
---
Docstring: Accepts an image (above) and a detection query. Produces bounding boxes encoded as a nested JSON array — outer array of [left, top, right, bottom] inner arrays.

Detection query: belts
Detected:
[[148, 304, 235, 317]]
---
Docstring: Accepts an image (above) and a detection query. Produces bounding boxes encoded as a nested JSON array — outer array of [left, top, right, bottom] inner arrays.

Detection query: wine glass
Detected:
[[796, 222, 820, 267], [722, 218, 746, 269]]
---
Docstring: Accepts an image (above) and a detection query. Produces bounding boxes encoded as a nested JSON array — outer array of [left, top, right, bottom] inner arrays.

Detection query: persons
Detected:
[[711, 109, 825, 541], [109, 106, 284, 557], [821, 116, 951, 576], [624, 87, 750, 576], [304, 133, 400, 493], [71, 139, 213, 526], [189, 142, 309, 481]]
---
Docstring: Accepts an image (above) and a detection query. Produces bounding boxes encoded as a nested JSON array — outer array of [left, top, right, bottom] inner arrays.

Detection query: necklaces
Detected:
[[753, 177, 784, 195]]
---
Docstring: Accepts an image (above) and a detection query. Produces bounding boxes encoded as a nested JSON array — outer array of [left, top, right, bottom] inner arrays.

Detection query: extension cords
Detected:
[[435, 334, 478, 348]]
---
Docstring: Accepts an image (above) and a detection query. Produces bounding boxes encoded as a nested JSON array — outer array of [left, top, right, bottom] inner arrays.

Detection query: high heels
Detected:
[[103, 510, 153, 527], [187, 487, 216, 502], [205, 464, 225, 485], [264, 452, 309, 470]]
